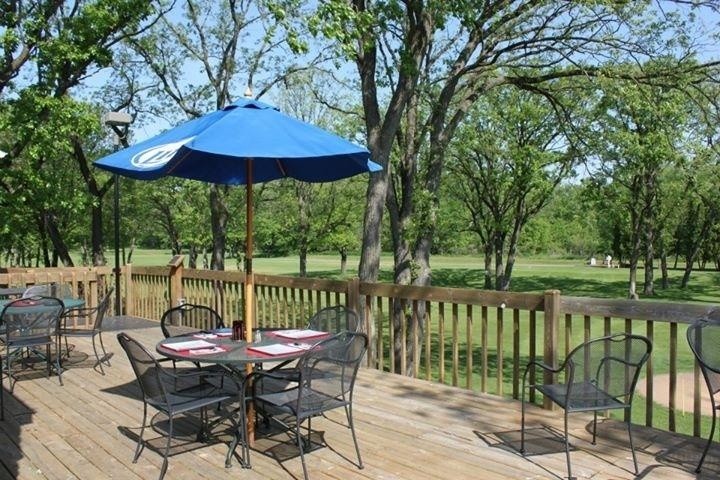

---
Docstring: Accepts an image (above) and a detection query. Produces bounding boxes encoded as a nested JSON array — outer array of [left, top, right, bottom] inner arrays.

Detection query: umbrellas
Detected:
[[90, 85, 384, 452]]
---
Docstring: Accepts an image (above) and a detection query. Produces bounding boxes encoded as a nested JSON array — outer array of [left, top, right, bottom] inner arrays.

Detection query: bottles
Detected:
[[254, 329, 262, 343]]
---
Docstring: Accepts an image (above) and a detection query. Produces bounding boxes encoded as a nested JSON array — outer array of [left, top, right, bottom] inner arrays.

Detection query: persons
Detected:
[[605, 253, 613, 268], [586, 256, 597, 266]]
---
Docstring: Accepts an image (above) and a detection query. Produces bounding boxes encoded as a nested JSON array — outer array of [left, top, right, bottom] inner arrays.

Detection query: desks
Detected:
[[0, 299, 88, 381], [158, 328, 342, 466]]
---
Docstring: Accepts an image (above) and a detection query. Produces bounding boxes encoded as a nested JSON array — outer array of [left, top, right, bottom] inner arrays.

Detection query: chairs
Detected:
[[52, 282, 116, 376], [683, 316, 719, 478], [255, 304, 366, 445], [21, 281, 74, 298], [2, 295, 72, 393], [113, 331, 258, 480], [156, 303, 253, 428], [245, 325, 375, 480], [509, 330, 655, 480]]
